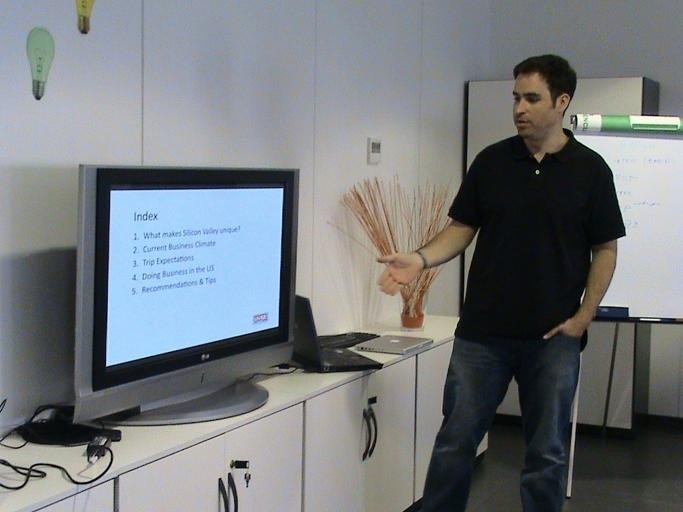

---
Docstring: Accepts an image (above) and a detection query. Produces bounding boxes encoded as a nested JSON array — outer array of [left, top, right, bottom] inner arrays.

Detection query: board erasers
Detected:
[[595, 306, 629, 319]]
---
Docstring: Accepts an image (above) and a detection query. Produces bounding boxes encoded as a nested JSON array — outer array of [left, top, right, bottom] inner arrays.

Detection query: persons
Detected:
[[377, 55, 626, 511]]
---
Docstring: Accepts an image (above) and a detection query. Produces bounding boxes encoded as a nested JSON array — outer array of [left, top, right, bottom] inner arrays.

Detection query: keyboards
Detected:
[[318, 333, 380, 348]]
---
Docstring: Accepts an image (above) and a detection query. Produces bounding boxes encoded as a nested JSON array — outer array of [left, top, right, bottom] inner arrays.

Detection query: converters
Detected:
[[87, 435, 111, 464]]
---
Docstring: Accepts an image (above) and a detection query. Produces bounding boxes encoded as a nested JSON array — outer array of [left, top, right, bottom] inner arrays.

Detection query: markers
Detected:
[[638, 318, 674, 323]]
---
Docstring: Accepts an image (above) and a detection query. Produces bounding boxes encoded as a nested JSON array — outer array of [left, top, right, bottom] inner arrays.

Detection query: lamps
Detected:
[[26, 26, 56, 100], [75, 0, 95, 34]]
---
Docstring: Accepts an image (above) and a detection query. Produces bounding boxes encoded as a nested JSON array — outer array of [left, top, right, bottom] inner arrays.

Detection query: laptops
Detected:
[[355, 335, 433, 354], [291, 293, 383, 373]]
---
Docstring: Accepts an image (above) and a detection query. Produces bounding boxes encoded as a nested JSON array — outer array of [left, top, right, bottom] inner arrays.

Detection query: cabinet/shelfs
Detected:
[[304, 339, 489, 511], [27, 480, 116, 512], [112, 403, 304, 511]]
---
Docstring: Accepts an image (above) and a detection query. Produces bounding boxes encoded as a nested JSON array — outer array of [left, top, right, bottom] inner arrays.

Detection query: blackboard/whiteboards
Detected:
[[572, 131, 683, 324]]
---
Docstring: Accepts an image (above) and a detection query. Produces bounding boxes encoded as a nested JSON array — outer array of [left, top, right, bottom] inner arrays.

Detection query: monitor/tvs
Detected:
[[74, 163, 300, 426]]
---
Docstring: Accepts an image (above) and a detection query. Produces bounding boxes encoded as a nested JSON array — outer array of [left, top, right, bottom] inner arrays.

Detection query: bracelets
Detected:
[[415, 250, 429, 267]]
